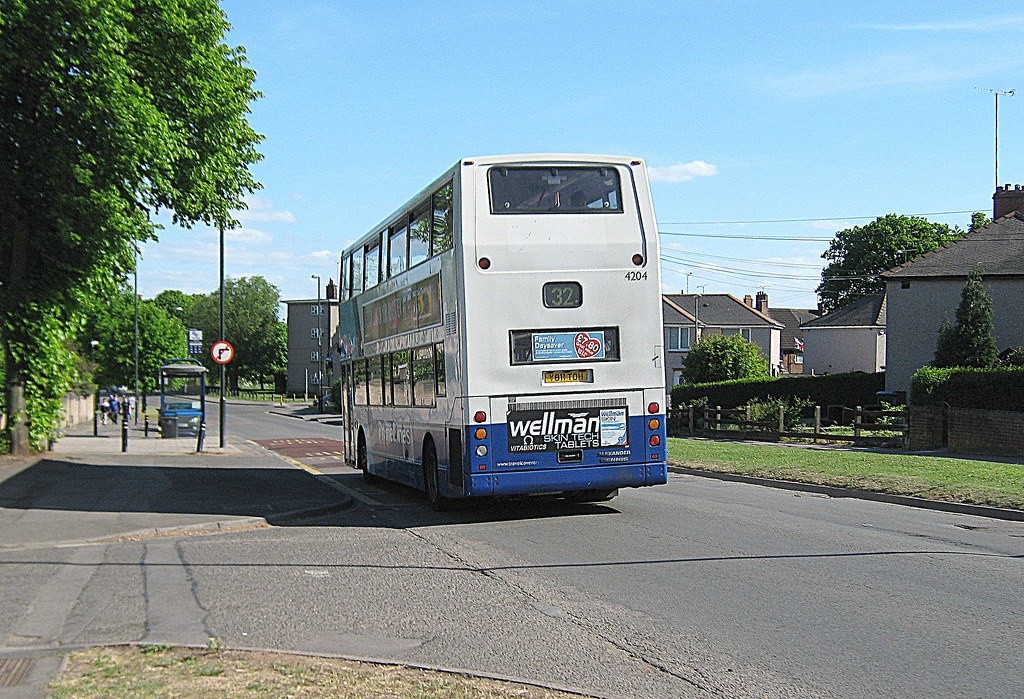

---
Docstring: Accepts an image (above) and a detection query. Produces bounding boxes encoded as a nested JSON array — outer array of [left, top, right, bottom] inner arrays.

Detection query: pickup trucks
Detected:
[[158, 401, 202, 432]]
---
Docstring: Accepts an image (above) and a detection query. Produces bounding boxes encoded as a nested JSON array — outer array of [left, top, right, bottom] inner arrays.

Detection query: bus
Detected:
[[341, 150, 670, 511]]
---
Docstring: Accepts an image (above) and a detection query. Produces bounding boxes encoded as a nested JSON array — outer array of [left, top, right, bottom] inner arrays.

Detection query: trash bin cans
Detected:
[[160, 410, 179, 440]]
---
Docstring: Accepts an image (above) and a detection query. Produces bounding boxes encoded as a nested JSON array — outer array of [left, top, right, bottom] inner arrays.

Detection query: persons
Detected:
[[99, 398, 111, 425], [128, 394, 135, 406], [121, 398, 132, 420], [571, 190, 588, 207], [108, 394, 120, 425]]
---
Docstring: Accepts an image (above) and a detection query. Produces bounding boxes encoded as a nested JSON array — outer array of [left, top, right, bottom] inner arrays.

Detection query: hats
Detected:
[[110, 395, 113, 397], [114, 394, 117, 397]]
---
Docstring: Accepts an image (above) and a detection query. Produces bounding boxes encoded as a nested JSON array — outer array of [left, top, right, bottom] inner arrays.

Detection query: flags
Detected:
[[794, 337, 804, 352]]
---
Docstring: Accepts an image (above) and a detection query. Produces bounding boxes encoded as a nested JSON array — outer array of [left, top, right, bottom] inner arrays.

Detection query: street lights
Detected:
[[694, 294, 703, 344], [312, 274, 324, 413]]
